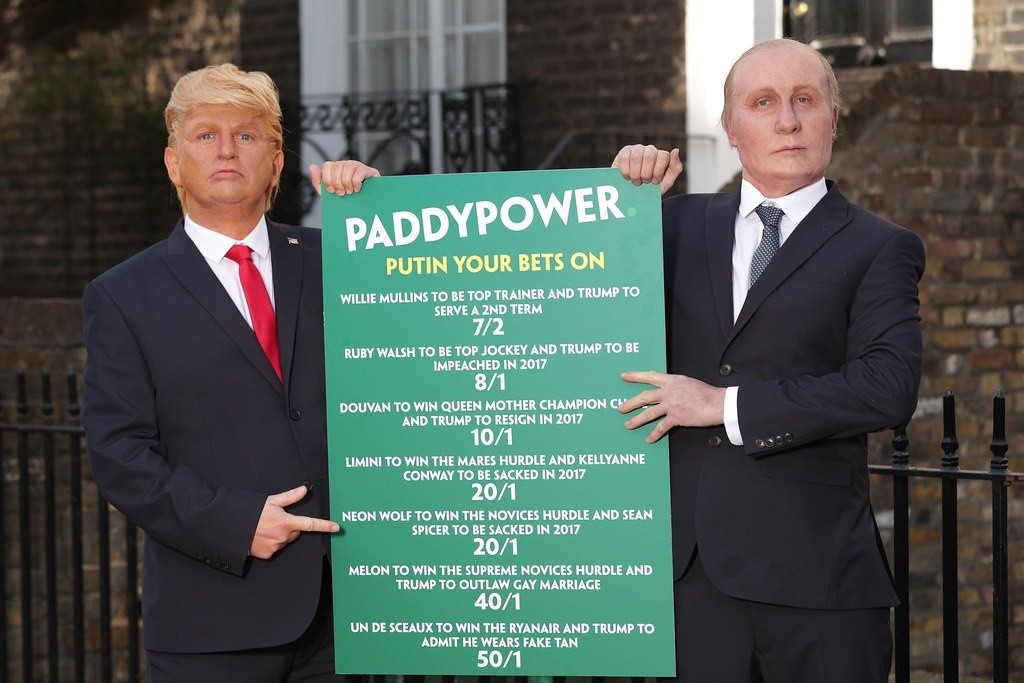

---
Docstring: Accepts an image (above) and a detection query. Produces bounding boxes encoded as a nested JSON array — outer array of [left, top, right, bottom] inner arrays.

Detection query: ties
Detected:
[[224, 244, 283, 383], [750, 205, 785, 290]]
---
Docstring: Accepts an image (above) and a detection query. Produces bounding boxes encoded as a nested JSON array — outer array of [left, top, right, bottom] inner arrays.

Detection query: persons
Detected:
[[83, 62, 381, 683], [608, 38, 927, 683]]
